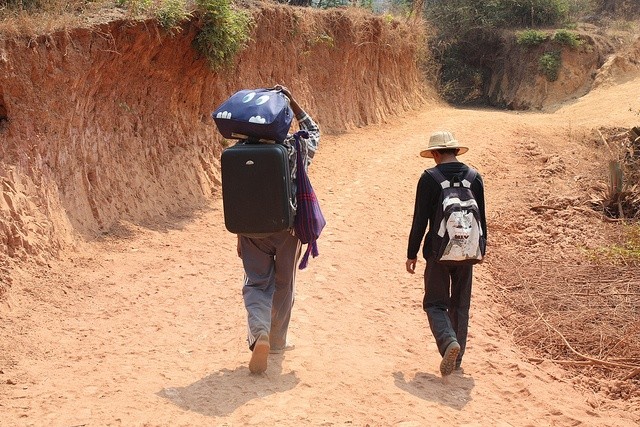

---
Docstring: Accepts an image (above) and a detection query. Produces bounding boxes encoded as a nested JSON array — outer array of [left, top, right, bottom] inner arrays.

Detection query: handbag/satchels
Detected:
[[294, 131, 326, 269], [212, 88, 294, 142]]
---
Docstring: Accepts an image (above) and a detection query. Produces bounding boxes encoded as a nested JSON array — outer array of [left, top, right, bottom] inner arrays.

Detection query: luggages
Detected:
[[221, 144, 291, 233]]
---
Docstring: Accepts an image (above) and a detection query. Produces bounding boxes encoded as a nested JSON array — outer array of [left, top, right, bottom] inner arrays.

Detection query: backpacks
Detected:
[[425, 166, 486, 265]]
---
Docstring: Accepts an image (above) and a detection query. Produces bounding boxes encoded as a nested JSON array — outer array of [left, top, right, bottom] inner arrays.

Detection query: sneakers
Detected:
[[440, 341, 461, 376]]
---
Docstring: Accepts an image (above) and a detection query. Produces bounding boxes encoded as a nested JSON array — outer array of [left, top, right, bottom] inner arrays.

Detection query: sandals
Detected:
[[248, 334, 271, 375], [269, 343, 294, 353]]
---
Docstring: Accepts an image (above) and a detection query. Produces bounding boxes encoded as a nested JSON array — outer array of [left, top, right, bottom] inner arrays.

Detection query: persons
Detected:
[[235, 83, 320, 375], [405, 130, 488, 373]]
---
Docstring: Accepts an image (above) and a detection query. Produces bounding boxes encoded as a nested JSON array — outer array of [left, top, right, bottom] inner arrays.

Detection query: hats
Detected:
[[419, 133, 469, 157]]
